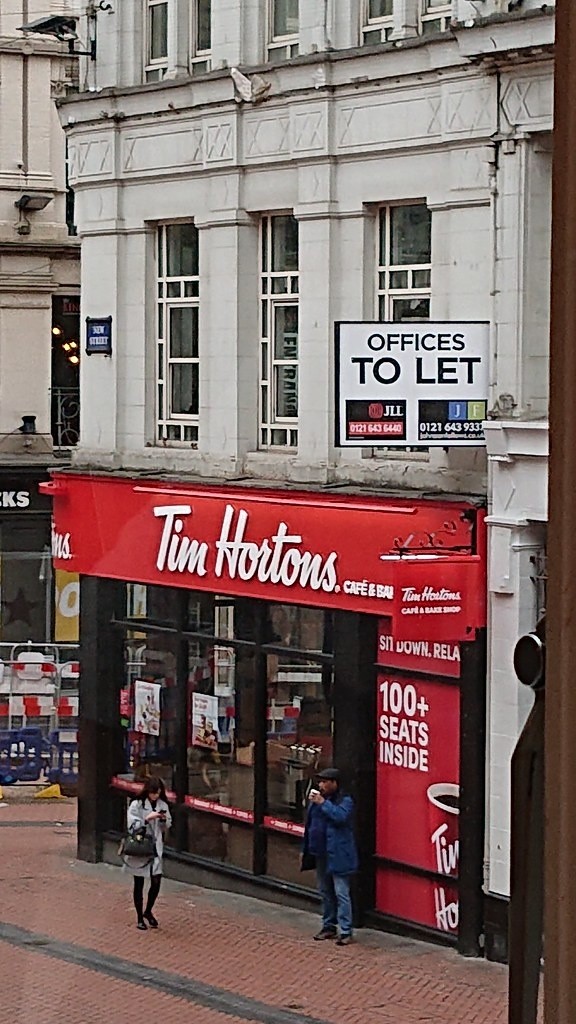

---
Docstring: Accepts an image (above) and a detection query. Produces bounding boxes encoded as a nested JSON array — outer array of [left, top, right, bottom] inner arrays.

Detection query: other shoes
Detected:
[[313, 928, 338, 940], [143, 911, 158, 926], [337, 934, 352, 945], [138, 915, 147, 929]]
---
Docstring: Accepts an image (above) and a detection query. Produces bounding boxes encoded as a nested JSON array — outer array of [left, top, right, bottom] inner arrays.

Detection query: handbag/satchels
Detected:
[[117, 819, 158, 858]]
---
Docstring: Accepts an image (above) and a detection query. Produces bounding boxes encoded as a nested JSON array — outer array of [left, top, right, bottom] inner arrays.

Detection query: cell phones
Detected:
[[159, 809, 167, 814]]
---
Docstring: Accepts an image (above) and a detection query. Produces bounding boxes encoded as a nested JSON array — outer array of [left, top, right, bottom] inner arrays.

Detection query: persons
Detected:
[[136, 695, 160, 735], [121, 776, 172, 931], [195, 714, 217, 747], [300, 768, 360, 945]]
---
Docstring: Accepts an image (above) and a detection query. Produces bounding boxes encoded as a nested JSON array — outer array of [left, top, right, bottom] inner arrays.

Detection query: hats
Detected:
[[314, 769, 343, 782]]
